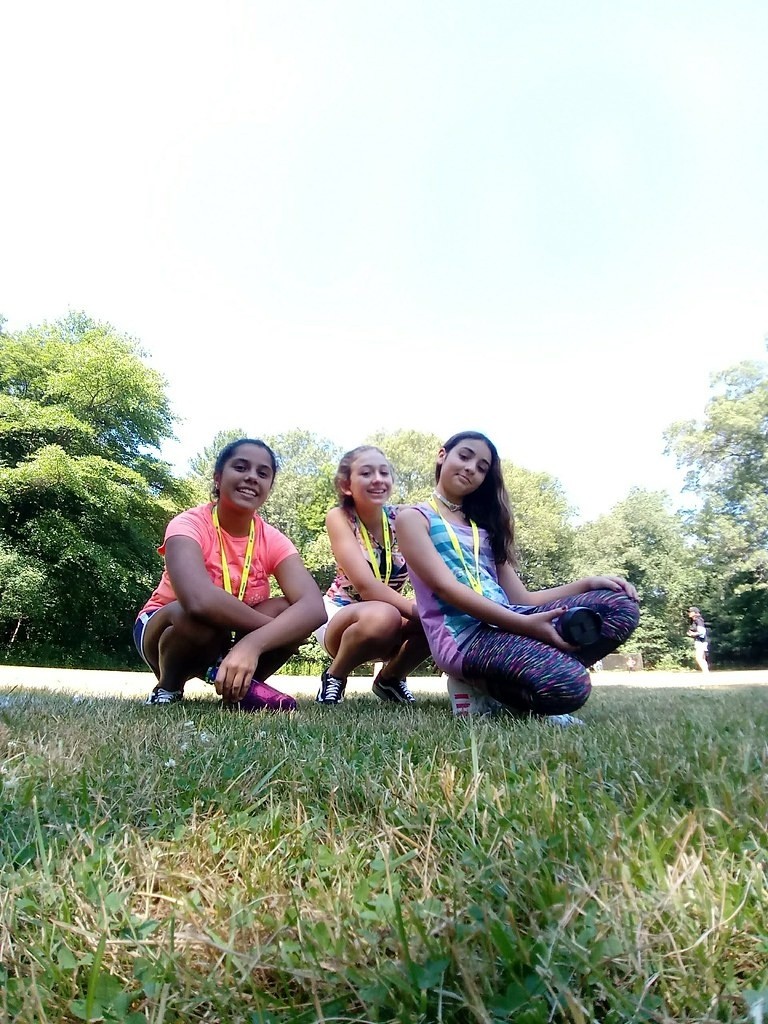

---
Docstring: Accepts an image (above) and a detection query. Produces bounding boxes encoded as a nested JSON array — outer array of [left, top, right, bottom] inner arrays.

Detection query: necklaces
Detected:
[[433, 490, 463, 511]]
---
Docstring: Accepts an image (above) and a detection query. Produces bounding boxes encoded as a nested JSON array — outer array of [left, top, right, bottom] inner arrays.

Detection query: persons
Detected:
[[686, 606, 710, 673], [312, 445, 422, 709], [133, 438, 328, 710], [394, 432, 641, 728]]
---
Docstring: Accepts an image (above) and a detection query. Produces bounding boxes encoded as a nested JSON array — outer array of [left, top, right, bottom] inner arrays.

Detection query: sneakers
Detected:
[[371, 670, 414, 707], [448, 674, 497, 719], [315, 665, 347, 707], [547, 713, 584, 725], [146, 680, 182, 705]]
